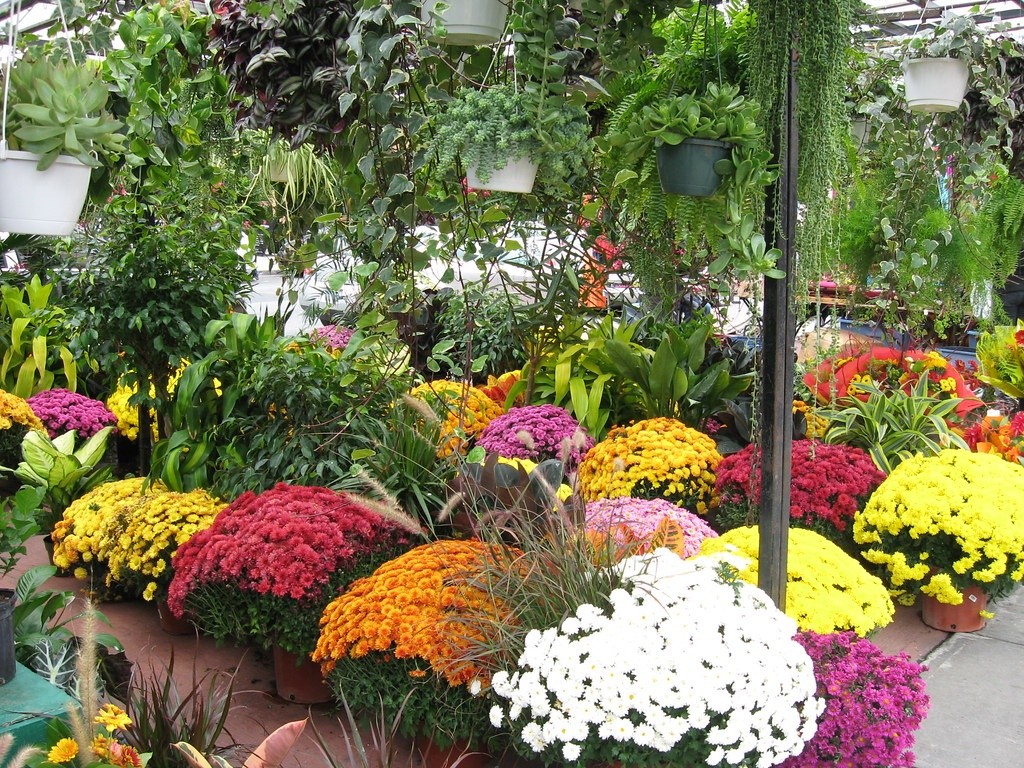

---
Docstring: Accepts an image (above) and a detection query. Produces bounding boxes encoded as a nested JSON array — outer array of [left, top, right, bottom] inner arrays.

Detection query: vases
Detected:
[[0, 442, 22, 498], [112, 422, 151, 480], [155, 592, 204, 637], [412, 716, 488, 768], [271, 633, 339, 703], [48, 357, 112, 403], [43, 532, 92, 578], [0, 588, 18, 688], [919, 565, 991, 632]]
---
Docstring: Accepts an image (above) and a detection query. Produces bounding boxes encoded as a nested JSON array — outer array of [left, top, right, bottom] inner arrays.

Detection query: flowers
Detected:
[[0, 342, 1024, 768]]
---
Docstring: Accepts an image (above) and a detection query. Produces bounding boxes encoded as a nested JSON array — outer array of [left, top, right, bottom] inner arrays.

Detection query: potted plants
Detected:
[[0, 0, 1024, 306]]
